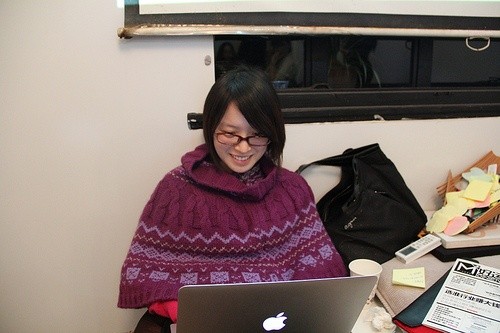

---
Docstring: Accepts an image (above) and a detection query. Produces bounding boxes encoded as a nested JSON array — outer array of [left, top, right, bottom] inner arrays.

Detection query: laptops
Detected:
[[171, 275, 378, 333]]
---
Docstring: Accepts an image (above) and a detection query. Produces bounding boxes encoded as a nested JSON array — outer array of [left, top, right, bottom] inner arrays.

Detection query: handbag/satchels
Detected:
[[296, 143, 426, 268]]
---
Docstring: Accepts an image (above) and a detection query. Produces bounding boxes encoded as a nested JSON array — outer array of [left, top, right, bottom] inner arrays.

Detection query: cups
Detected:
[[348, 259, 383, 305]]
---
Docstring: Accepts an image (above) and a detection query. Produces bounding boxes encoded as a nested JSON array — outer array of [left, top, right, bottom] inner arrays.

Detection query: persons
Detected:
[[117, 66, 348, 333], [216, 41, 236, 61]]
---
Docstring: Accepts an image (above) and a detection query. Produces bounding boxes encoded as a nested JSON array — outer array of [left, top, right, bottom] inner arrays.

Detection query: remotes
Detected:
[[394, 234, 442, 262]]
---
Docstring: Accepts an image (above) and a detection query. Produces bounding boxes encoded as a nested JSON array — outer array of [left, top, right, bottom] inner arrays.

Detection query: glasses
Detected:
[[214, 129, 273, 146]]
